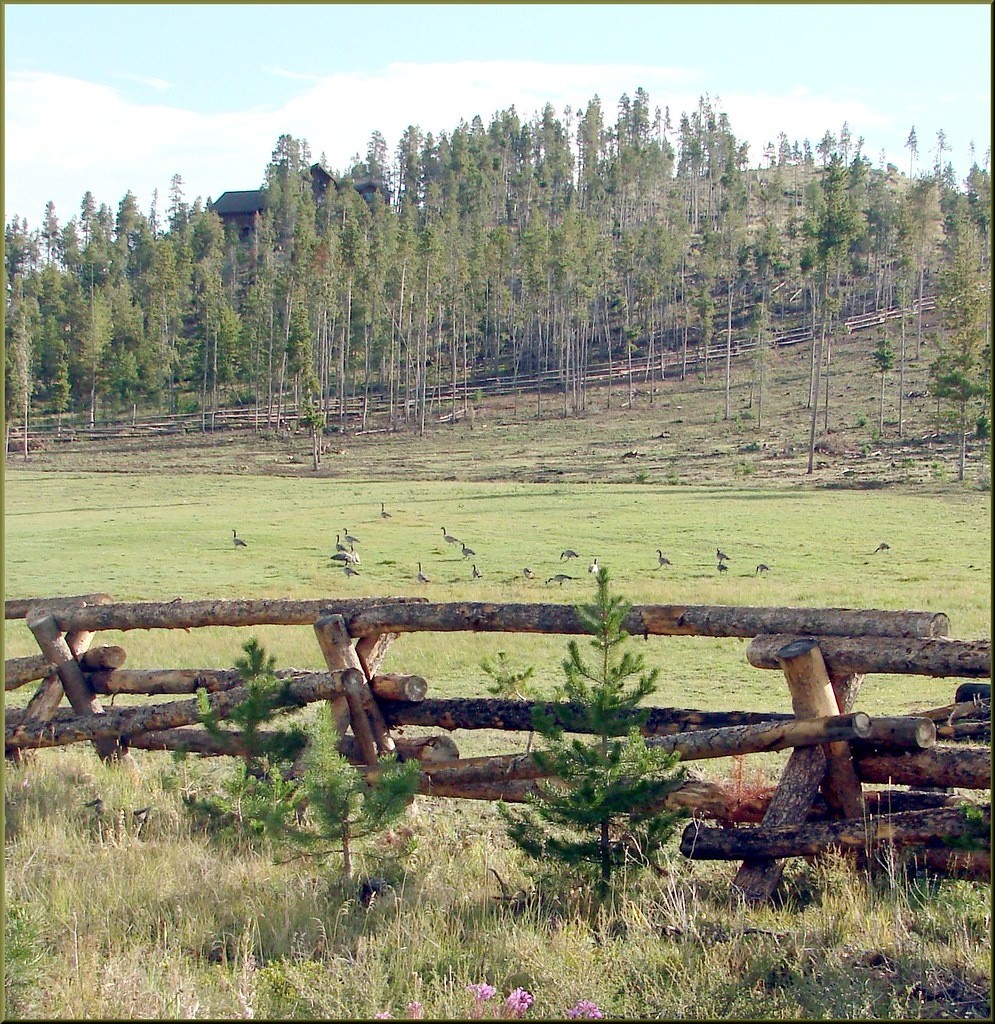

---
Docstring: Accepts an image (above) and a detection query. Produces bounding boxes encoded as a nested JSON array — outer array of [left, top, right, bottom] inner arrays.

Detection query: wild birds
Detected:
[[471, 565, 483, 580], [231, 529, 247, 549], [335, 535, 349, 553], [381, 503, 393, 519], [656, 549, 673, 568], [560, 549, 579, 562], [523, 567, 533, 577], [873, 544, 891, 554], [440, 526, 461, 547], [587, 559, 598, 573], [717, 561, 728, 575], [343, 527, 361, 546], [348, 544, 360, 566], [716, 548, 731, 563], [330, 552, 363, 567], [460, 542, 475, 560], [756, 564, 769, 574], [343, 556, 361, 579], [545, 574, 573, 586], [415, 562, 431, 584]]
